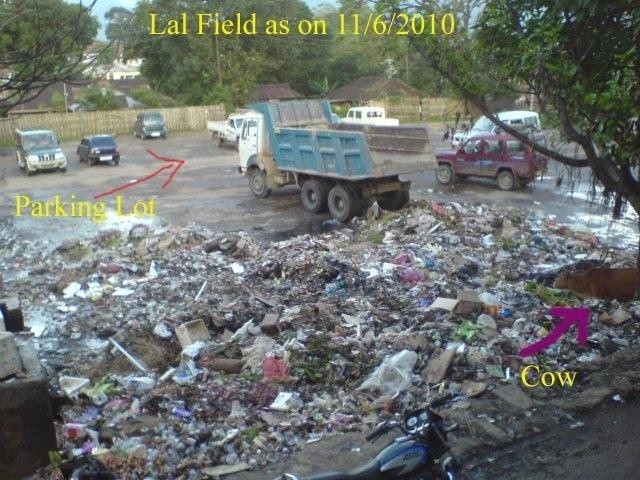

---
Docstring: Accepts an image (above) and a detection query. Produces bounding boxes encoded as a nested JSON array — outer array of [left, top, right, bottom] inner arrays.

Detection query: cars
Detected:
[[133, 111, 168, 140], [76, 135, 120, 166], [435, 110, 550, 191]]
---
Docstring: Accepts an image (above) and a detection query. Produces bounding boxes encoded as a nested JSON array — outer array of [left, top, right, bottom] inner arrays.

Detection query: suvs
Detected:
[[14, 126, 67, 176]]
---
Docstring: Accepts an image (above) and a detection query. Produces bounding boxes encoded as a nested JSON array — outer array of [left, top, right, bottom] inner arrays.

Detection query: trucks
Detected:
[[238, 99, 439, 223]]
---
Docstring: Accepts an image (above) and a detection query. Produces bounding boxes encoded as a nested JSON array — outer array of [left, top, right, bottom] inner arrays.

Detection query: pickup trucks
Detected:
[[207, 113, 244, 148], [332, 106, 399, 126]]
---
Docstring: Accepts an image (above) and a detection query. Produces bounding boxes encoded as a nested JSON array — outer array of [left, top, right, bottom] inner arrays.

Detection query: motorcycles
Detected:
[[268, 392, 472, 480]]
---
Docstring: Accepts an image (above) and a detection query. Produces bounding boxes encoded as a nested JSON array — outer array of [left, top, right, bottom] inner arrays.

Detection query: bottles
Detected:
[[61, 423, 87, 438]]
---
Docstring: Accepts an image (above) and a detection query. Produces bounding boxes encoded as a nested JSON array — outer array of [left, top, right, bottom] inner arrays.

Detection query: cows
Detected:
[[552, 267, 640, 304]]
